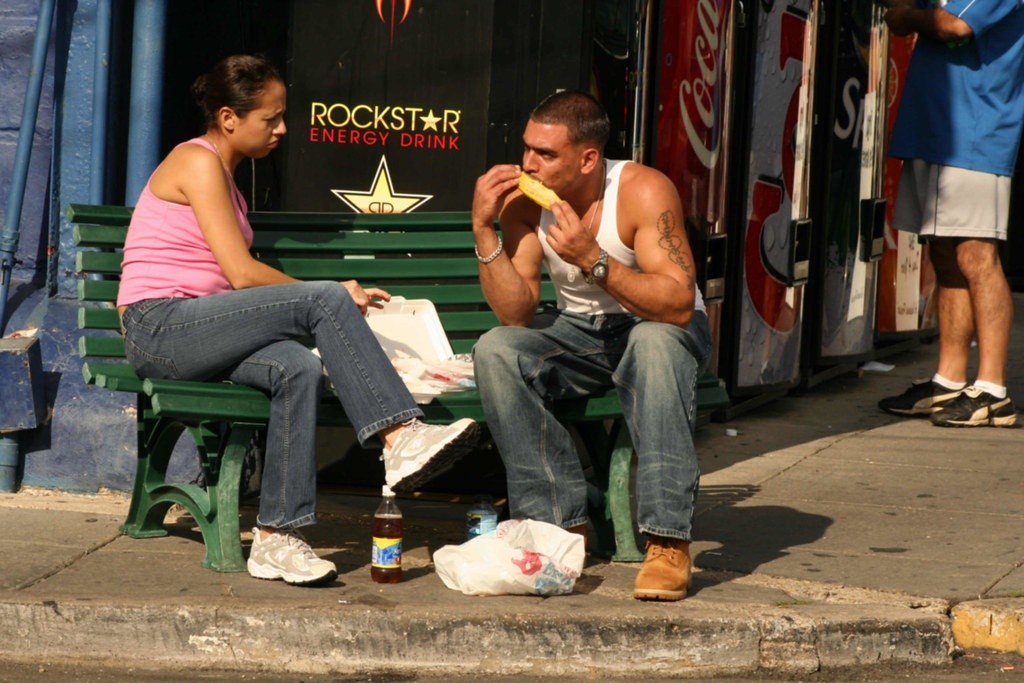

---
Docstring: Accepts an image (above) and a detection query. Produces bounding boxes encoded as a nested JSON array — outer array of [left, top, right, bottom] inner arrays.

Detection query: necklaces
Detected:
[[205, 131, 237, 192], [567, 164, 606, 284]]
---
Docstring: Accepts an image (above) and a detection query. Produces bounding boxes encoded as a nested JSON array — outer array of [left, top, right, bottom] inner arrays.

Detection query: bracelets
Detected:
[[474, 234, 502, 265]]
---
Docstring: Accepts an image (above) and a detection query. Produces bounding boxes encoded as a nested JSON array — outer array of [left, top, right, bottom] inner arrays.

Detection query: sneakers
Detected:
[[380, 417, 477, 490], [634, 532, 692, 600], [929, 386, 1016, 427], [248, 525, 337, 587], [878, 377, 969, 417], [568, 524, 588, 558]]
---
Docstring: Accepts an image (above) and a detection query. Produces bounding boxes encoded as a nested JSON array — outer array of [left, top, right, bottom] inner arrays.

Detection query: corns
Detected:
[[517, 171, 560, 211]]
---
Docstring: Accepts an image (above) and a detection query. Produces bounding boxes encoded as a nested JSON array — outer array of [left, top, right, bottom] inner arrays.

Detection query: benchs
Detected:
[[66, 203, 730, 573]]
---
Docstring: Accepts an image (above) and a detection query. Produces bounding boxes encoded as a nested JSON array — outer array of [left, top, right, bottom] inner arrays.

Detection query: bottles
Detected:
[[467, 494, 498, 541], [370, 484, 401, 583]]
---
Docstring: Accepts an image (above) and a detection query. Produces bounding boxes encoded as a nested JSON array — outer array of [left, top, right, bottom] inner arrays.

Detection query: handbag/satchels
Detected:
[[433, 514, 587, 595]]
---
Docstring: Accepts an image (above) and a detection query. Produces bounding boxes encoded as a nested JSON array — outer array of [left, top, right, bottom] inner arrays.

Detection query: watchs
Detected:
[[581, 247, 606, 282]]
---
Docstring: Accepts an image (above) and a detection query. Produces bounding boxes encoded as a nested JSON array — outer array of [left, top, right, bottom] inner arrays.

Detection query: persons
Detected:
[[118, 52, 484, 584], [432, 92, 709, 601], [878, 0, 1024, 428]]
